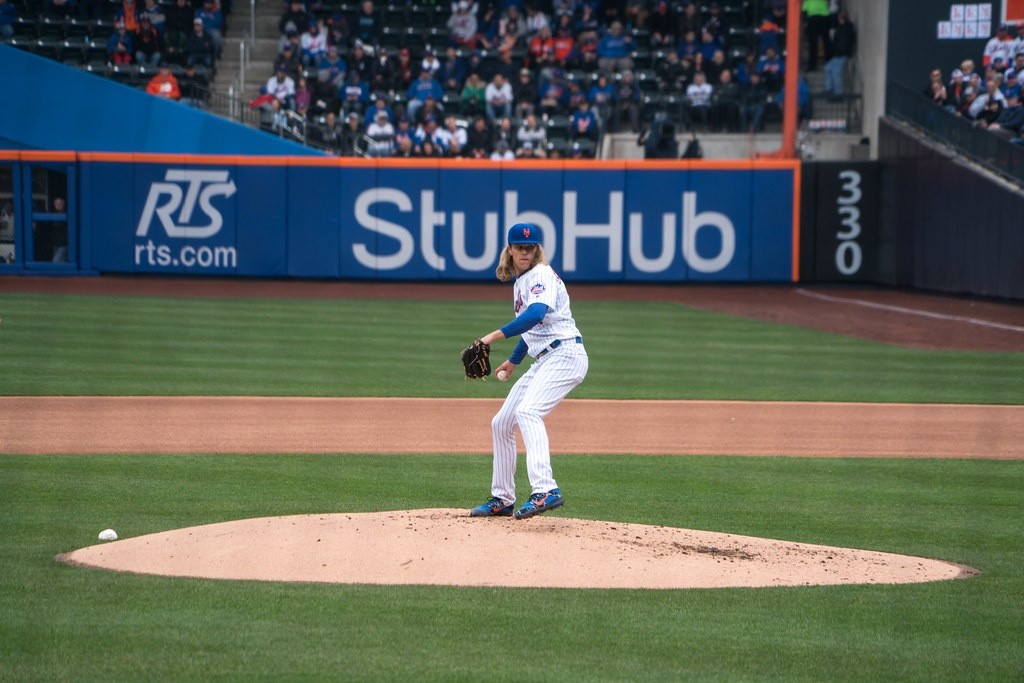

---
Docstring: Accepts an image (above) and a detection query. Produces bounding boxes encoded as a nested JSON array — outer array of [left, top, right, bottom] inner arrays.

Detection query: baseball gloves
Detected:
[[460, 338, 491, 382]]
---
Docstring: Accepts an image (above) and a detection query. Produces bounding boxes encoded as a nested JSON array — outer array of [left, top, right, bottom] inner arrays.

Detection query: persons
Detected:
[[921, 21, 1024, 148], [103, 0, 854, 161], [461, 222, 588, 518]]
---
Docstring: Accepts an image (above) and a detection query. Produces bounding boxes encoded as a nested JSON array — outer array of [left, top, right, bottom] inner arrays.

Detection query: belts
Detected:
[[537, 337, 582, 360]]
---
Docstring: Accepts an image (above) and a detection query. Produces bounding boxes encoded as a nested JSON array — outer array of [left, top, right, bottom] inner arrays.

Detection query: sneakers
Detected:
[[469, 496, 515, 516], [514, 488, 564, 520]]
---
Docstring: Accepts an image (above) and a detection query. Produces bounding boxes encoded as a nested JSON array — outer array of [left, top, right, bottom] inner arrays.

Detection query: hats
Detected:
[[507, 222, 544, 244]]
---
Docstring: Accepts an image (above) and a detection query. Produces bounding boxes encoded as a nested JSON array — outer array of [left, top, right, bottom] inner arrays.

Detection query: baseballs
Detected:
[[497, 370, 508, 382]]
